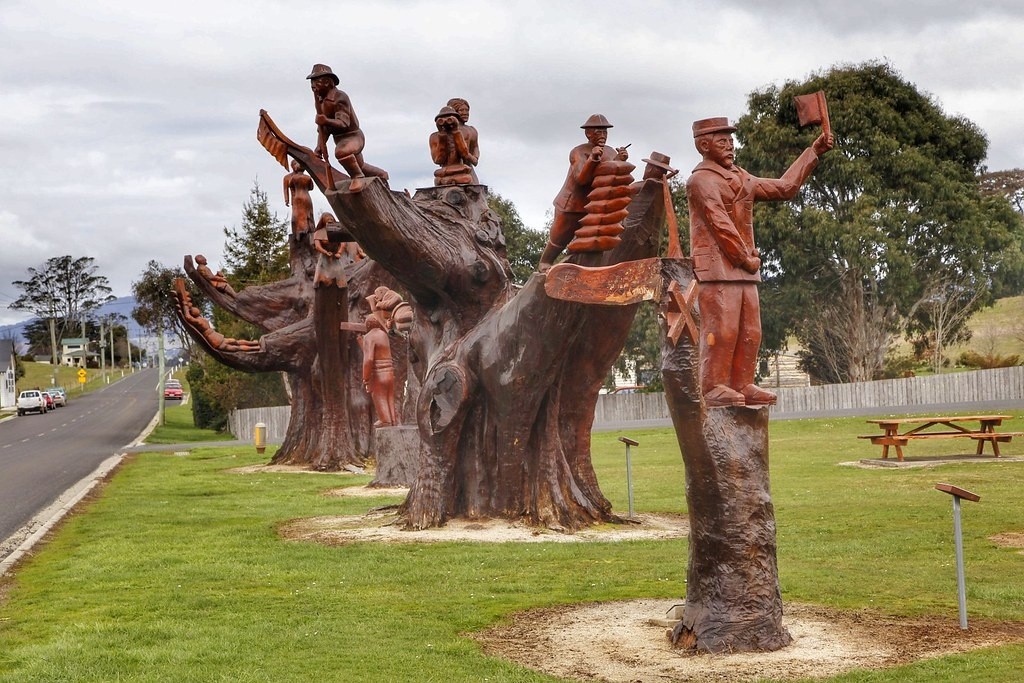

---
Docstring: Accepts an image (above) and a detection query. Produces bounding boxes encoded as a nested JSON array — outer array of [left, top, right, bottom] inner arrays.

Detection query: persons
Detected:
[[373, 287, 413, 329], [306, 63, 389, 193], [361, 313, 399, 428], [196, 254, 245, 300], [686, 117, 833, 411], [537, 111, 629, 272], [284, 160, 369, 290], [180, 300, 261, 352], [428, 97, 481, 185]]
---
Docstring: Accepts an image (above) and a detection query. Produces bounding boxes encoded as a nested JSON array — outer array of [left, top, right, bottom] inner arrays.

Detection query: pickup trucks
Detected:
[[16, 389, 48, 416]]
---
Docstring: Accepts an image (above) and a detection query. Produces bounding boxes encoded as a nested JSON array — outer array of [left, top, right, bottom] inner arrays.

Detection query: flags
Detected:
[[796, 90, 829, 124]]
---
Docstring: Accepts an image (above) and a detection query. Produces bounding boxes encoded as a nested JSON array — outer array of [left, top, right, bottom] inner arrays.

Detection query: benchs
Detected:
[[856, 429, 1024, 462]]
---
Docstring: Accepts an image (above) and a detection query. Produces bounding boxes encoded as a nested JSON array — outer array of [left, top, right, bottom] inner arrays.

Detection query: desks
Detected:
[[865, 415, 1016, 462]]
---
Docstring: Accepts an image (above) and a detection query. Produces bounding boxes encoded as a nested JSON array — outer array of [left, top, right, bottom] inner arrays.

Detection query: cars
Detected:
[[45, 390, 65, 407], [167, 378, 179, 383], [39, 392, 56, 410], [50, 387, 68, 402], [164, 383, 184, 399]]
